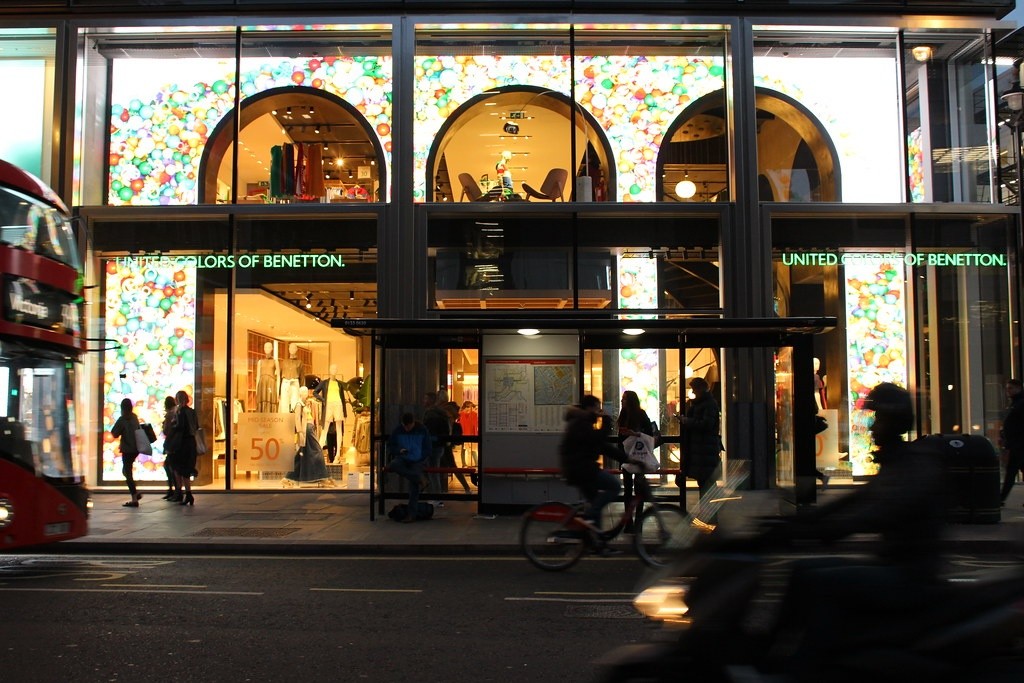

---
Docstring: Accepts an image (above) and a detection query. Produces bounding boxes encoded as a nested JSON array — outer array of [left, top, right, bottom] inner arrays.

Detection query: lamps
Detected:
[[272, 106, 353, 182], [649, 244, 712, 259], [500, 117, 535, 198], [275, 291, 378, 318], [662, 169, 709, 203]]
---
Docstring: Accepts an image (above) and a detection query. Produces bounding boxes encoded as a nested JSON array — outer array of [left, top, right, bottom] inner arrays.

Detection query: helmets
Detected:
[[861, 382, 912, 412]]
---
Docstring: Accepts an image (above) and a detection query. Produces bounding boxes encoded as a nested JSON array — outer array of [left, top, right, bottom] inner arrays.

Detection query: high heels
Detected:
[[181, 491, 195, 506], [172, 490, 184, 502]]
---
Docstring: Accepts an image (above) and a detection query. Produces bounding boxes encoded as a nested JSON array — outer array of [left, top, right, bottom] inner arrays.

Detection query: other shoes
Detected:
[[137, 492, 142, 499], [122, 501, 139, 507], [573, 512, 604, 534], [420, 477, 431, 493], [622, 527, 642, 538], [400, 516, 413, 524], [164, 489, 177, 501], [597, 547, 628, 556]]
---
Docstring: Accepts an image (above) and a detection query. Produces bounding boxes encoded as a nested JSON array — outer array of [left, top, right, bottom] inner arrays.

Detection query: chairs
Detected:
[[522, 168, 568, 202], [458, 173, 490, 203]]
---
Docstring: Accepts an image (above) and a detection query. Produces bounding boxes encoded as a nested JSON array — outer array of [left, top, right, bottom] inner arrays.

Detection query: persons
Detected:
[[386, 392, 479, 503], [615, 391, 651, 533], [497, 151, 513, 202], [161, 390, 198, 506], [998, 378, 1024, 504], [111, 398, 141, 507], [312, 365, 348, 463], [281, 386, 330, 486], [678, 378, 721, 525], [255, 342, 304, 413], [749, 382, 948, 683], [813, 358, 826, 415], [556, 394, 645, 542]]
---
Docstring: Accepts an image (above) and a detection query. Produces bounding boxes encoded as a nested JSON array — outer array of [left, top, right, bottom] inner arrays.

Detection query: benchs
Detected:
[[484, 467, 681, 479], [383, 466, 476, 477]]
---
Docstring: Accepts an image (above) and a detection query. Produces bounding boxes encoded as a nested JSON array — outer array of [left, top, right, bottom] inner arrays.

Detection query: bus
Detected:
[[0, 159, 127, 552]]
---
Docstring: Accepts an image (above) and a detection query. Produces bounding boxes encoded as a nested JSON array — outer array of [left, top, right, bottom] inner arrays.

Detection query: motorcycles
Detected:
[[591, 459, 1024, 683]]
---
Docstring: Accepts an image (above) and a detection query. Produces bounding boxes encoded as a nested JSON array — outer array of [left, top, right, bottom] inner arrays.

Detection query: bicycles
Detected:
[[520, 460, 695, 570]]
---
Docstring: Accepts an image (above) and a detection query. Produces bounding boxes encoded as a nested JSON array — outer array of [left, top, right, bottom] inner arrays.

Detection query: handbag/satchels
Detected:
[[623, 433, 661, 476], [134, 424, 153, 456], [141, 423, 157, 443], [194, 427, 208, 455]]
